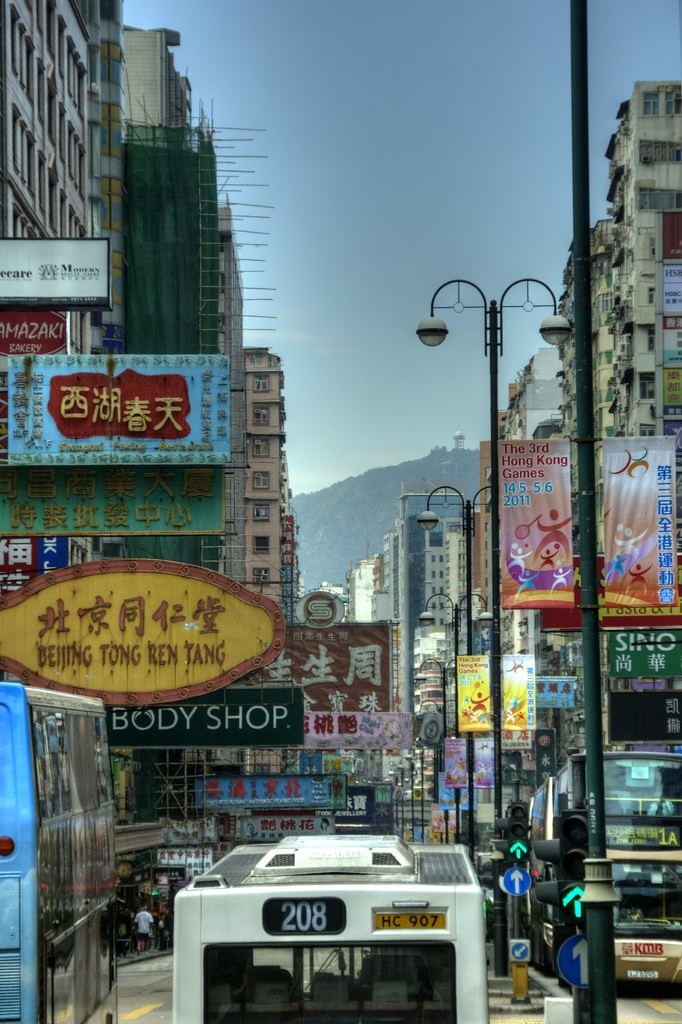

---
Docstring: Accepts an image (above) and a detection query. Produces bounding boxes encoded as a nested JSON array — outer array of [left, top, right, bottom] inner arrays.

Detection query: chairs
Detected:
[[213, 969, 452, 1024]]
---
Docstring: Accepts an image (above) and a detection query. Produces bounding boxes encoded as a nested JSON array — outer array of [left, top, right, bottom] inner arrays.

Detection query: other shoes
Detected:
[[137, 948, 141, 955]]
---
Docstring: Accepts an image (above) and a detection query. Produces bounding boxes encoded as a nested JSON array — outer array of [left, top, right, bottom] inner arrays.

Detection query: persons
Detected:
[[117, 897, 172, 957]]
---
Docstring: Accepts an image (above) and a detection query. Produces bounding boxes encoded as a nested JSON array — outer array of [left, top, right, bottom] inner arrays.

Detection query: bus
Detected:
[[526, 751, 682, 986], [173, 833, 489, 1024], [1, 680, 133, 1024]]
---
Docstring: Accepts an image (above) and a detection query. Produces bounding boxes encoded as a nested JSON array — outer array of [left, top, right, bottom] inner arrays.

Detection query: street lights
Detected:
[[416, 700, 443, 803], [418, 592, 494, 862], [416, 281, 571, 973], [357, 747, 428, 842], [418, 484, 494, 866]]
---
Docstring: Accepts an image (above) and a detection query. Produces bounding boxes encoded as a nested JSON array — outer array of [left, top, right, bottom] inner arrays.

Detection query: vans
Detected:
[[473, 851, 495, 886]]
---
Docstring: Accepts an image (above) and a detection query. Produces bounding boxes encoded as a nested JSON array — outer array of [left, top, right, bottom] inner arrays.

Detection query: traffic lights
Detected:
[[533, 809, 588, 929], [495, 817, 508, 857], [507, 802, 529, 861]]
